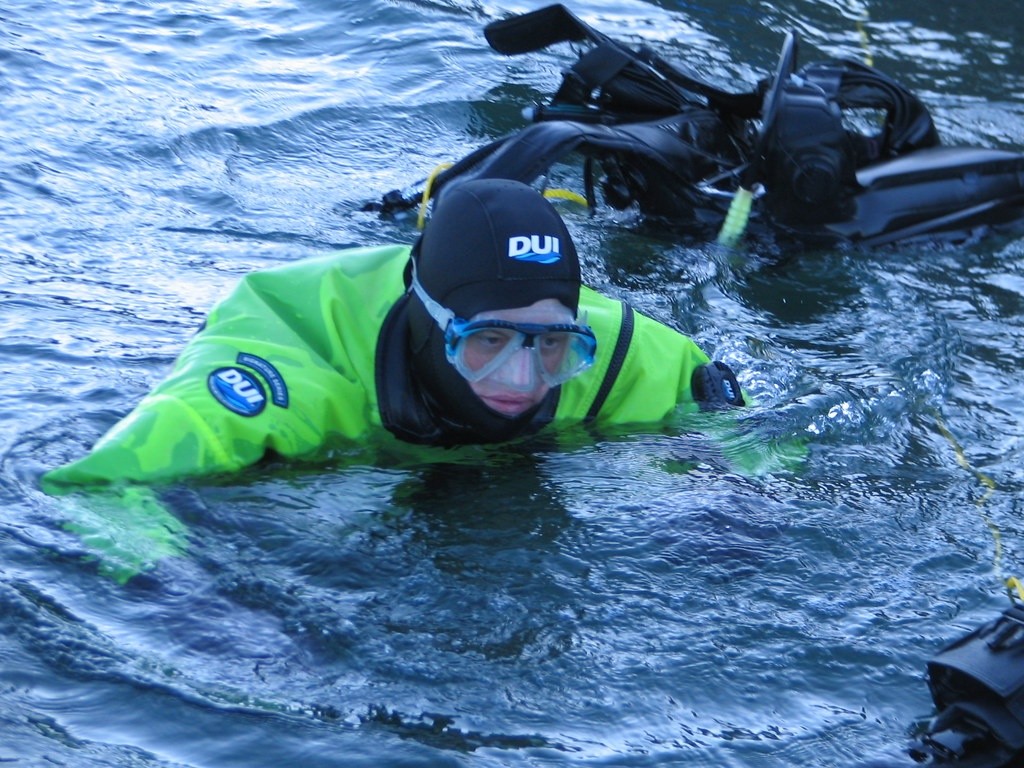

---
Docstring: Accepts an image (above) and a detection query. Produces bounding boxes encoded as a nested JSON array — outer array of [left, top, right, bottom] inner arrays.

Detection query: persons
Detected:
[[61, 178, 746, 485]]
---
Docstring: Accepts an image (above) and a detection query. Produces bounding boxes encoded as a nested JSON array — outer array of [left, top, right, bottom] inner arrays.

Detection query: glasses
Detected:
[[444, 318, 597, 391]]
